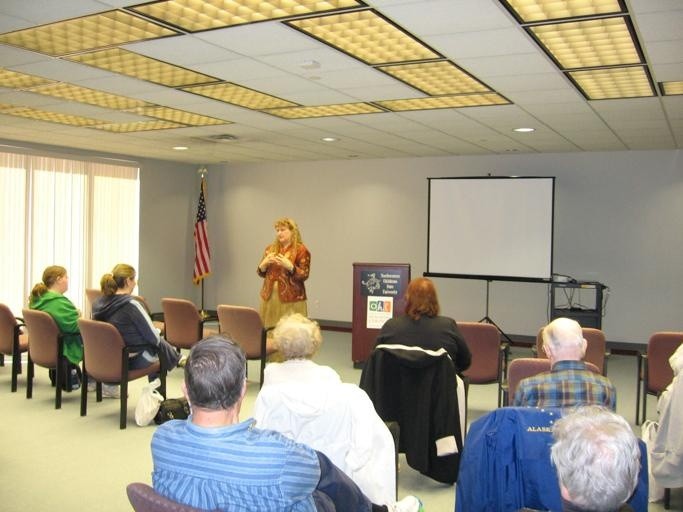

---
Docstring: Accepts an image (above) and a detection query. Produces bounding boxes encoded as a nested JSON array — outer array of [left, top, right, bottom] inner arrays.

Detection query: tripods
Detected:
[[479, 280, 514, 346]]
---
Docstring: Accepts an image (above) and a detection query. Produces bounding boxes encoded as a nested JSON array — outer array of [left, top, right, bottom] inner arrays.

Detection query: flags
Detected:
[[191, 166, 210, 289]]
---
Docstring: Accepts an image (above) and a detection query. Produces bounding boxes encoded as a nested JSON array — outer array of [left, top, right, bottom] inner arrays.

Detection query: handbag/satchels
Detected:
[[50, 364, 81, 390], [642, 420, 665, 502], [134, 378, 164, 426], [154, 397, 190, 425]]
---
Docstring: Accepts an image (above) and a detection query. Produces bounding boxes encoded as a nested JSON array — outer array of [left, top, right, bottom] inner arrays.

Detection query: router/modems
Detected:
[[552, 274, 573, 283]]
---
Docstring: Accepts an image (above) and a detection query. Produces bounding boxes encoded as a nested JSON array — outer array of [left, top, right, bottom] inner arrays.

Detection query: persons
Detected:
[[149, 337, 421, 511], [376, 278, 473, 408], [253, 317, 399, 492], [535, 404, 638, 511], [89, 260, 188, 404], [20, 266, 82, 381], [255, 216, 313, 334], [514, 317, 618, 407], [646, 332, 683, 495]]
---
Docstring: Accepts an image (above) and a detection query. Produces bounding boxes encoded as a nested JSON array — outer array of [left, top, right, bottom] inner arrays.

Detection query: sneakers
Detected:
[[383, 495, 424, 512], [87, 380, 120, 398]]
